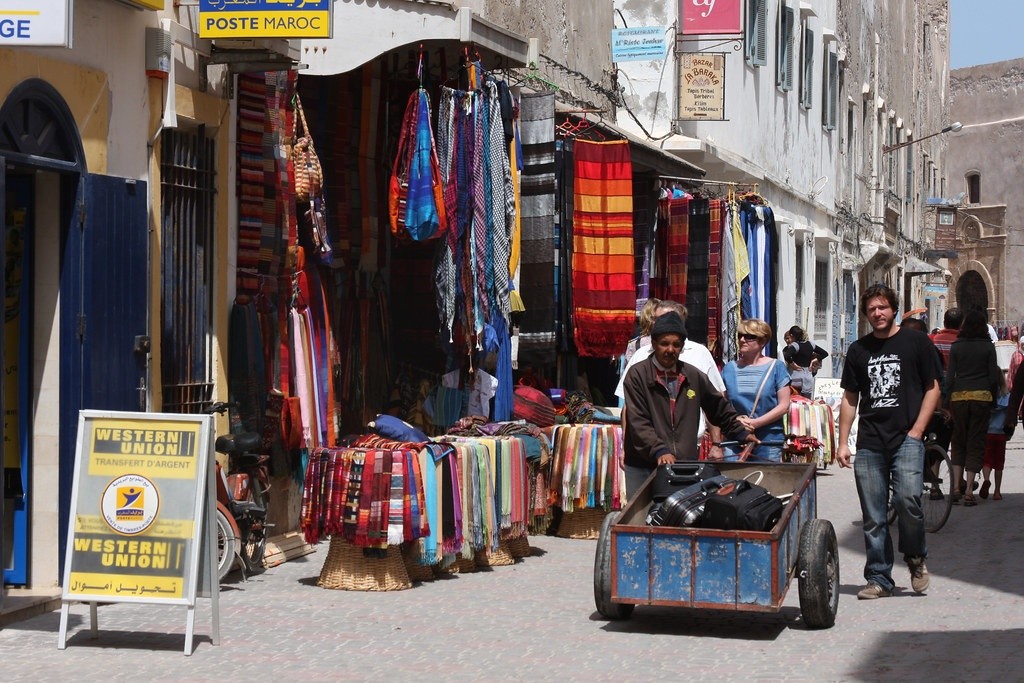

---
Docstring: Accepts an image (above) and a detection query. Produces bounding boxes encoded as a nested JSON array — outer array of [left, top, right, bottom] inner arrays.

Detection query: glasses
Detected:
[[737, 331, 758, 341]]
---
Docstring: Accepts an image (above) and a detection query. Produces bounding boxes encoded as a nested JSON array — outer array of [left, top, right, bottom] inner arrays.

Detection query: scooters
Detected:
[[201, 397, 271, 591]]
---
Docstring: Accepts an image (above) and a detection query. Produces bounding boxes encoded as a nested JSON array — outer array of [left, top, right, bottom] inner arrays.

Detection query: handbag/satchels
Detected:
[[389, 89, 446, 244], [304, 210, 332, 255], [290, 95, 322, 201]]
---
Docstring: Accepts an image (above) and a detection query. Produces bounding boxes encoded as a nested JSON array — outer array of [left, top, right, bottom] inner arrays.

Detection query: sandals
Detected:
[[965, 494, 976, 507], [930, 487, 945, 500], [953, 487, 961, 503]]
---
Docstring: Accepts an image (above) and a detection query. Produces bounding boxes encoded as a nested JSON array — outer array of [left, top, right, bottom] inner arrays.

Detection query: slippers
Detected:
[[994, 492, 1001, 501], [979, 478, 991, 499]]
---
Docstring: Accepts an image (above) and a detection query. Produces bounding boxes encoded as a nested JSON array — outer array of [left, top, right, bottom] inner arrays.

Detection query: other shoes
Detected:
[[923, 469, 944, 484], [961, 481, 979, 492]]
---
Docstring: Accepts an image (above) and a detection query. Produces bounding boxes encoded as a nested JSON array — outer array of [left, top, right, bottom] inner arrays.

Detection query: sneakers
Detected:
[[906, 556, 929, 591], [858, 579, 890, 598]]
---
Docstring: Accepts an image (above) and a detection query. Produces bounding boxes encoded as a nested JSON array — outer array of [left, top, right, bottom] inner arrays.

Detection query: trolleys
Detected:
[[592, 436, 841, 629]]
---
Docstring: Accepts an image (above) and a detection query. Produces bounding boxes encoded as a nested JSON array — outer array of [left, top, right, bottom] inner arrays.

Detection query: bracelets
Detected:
[[712, 442, 721, 447]]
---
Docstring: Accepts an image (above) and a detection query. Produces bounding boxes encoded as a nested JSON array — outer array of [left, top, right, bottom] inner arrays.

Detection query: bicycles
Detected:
[[886, 410, 956, 533]]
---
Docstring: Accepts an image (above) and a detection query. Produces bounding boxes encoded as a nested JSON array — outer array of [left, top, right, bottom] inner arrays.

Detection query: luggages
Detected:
[[645, 461, 783, 533]]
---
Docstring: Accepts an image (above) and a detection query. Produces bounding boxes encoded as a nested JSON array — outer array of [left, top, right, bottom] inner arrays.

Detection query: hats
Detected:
[[651, 311, 688, 340]]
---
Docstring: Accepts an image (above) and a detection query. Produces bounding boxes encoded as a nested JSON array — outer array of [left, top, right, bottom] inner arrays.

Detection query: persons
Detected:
[[781, 325, 828, 398], [834, 283, 943, 600], [706, 320, 792, 462], [616, 298, 762, 504], [899, 308, 1024, 505]]
